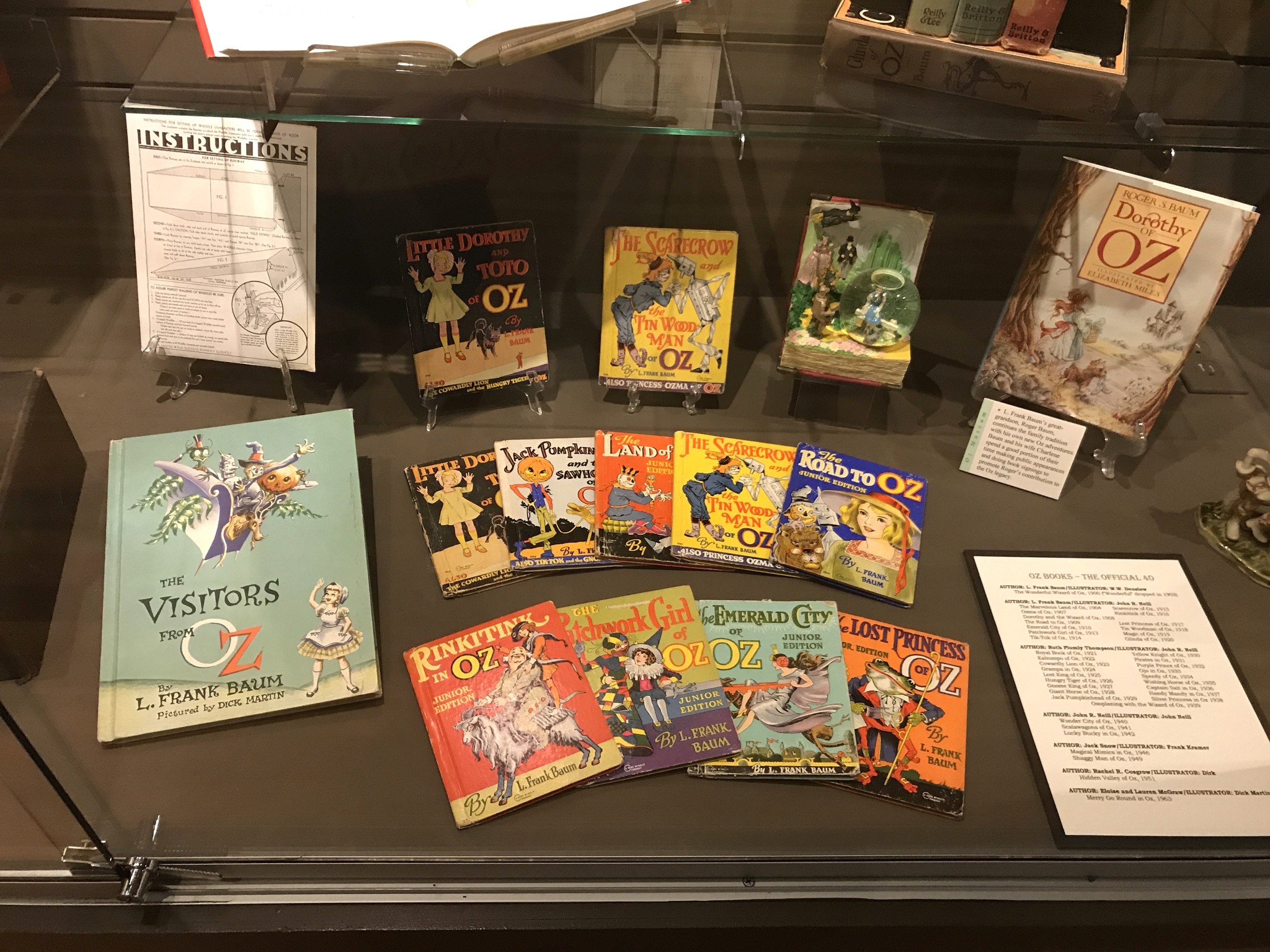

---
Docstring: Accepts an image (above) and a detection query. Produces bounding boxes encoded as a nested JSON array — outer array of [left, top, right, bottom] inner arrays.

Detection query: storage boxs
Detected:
[[0, 368, 87, 685]]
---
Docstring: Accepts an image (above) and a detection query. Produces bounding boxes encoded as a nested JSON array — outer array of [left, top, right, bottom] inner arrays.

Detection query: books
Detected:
[[99, 0, 1261, 832]]
